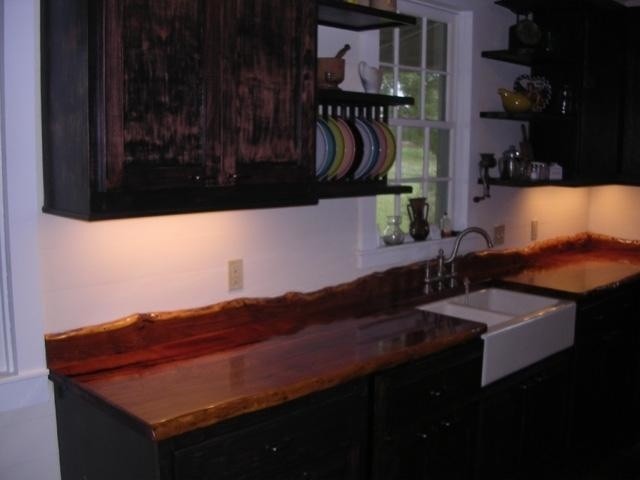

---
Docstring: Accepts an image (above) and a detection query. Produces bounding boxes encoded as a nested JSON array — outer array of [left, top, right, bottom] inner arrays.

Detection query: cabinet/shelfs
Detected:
[[473, 272, 639, 477], [479, 0, 579, 189], [40, 0, 318, 223], [319, 0, 416, 199], [52, 340, 477, 477], [527, 0, 640, 188]]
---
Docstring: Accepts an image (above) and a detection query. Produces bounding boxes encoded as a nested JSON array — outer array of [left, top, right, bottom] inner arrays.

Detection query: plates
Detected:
[[315, 114, 398, 184]]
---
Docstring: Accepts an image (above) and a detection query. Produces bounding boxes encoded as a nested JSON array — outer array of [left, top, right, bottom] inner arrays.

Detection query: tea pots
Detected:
[[499, 73, 536, 114]]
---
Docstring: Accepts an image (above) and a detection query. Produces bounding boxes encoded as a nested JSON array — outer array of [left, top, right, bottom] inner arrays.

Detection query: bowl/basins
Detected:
[[316, 56, 346, 91]]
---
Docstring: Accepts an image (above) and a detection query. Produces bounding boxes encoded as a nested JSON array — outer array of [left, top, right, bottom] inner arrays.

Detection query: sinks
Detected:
[[415, 303, 515, 390], [447, 284, 575, 357]]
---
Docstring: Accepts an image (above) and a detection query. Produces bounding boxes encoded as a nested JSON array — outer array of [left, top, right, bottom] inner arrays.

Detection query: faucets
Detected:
[[437, 224, 495, 292]]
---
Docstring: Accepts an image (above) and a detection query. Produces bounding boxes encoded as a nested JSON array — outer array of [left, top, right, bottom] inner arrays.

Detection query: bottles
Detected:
[[439, 211, 452, 238], [382, 216, 405, 245]]
